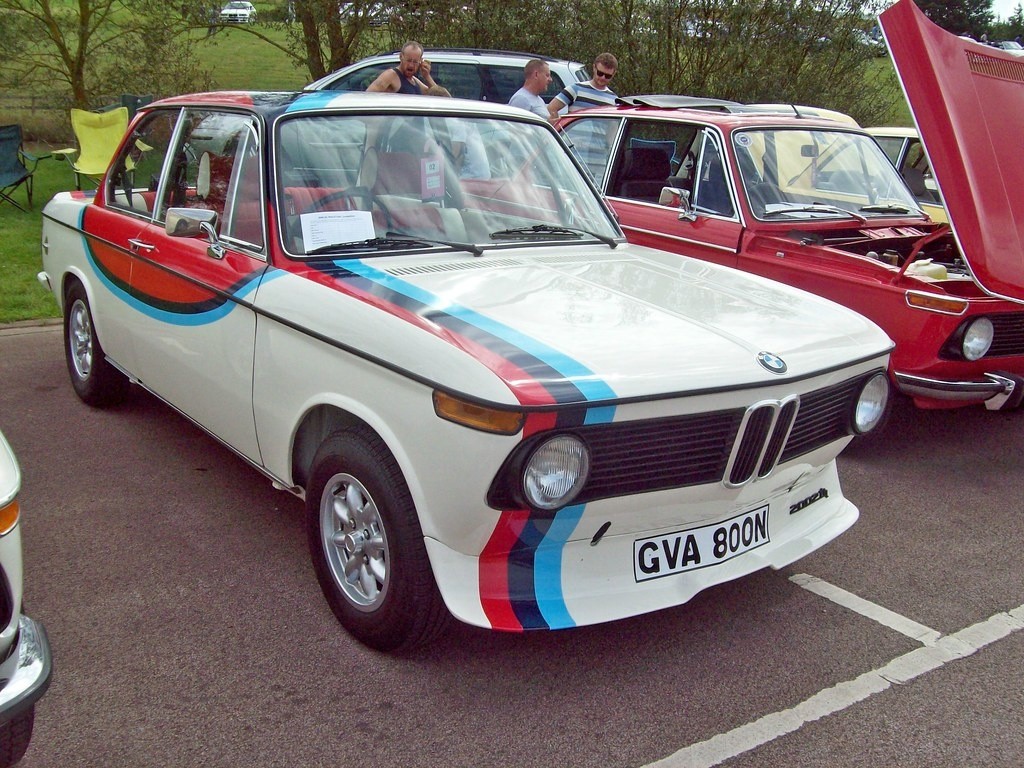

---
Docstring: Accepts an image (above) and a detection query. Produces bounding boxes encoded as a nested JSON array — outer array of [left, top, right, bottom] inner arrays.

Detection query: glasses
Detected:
[[403, 54, 422, 65], [595, 65, 614, 79]]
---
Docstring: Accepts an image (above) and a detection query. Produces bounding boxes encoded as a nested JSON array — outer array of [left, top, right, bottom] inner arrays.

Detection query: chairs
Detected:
[[99, 93, 150, 119], [370, 154, 467, 245], [899, 169, 935, 203], [53, 107, 153, 192], [189, 152, 291, 247], [618, 148, 675, 202], [0, 123, 52, 214]]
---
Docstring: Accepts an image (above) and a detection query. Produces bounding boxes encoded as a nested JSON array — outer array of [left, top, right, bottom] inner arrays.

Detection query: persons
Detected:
[[981, 30, 988, 42], [182, 4, 189, 19], [423, 85, 491, 179], [871, 22, 879, 40], [207, 6, 219, 36], [1015, 34, 1024, 47], [963, 29, 974, 36], [199, 6, 205, 22], [286, 2, 296, 24], [507, 59, 562, 161], [350, 40, 436, 186], [547, 53, 619, 192]]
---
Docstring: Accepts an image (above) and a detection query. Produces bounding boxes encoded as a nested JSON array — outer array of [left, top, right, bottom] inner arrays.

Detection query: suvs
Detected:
[[284, 48, 590, 164]]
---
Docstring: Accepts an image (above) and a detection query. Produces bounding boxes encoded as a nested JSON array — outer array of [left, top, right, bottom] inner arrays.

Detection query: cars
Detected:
[[219, 1, 258, 25], [618, 11, 1024, 68], [341, 1, 483, 27], [1, 432, 54, 768], [439, 0, 1023, 431], [36, 88, 897, 660]]
